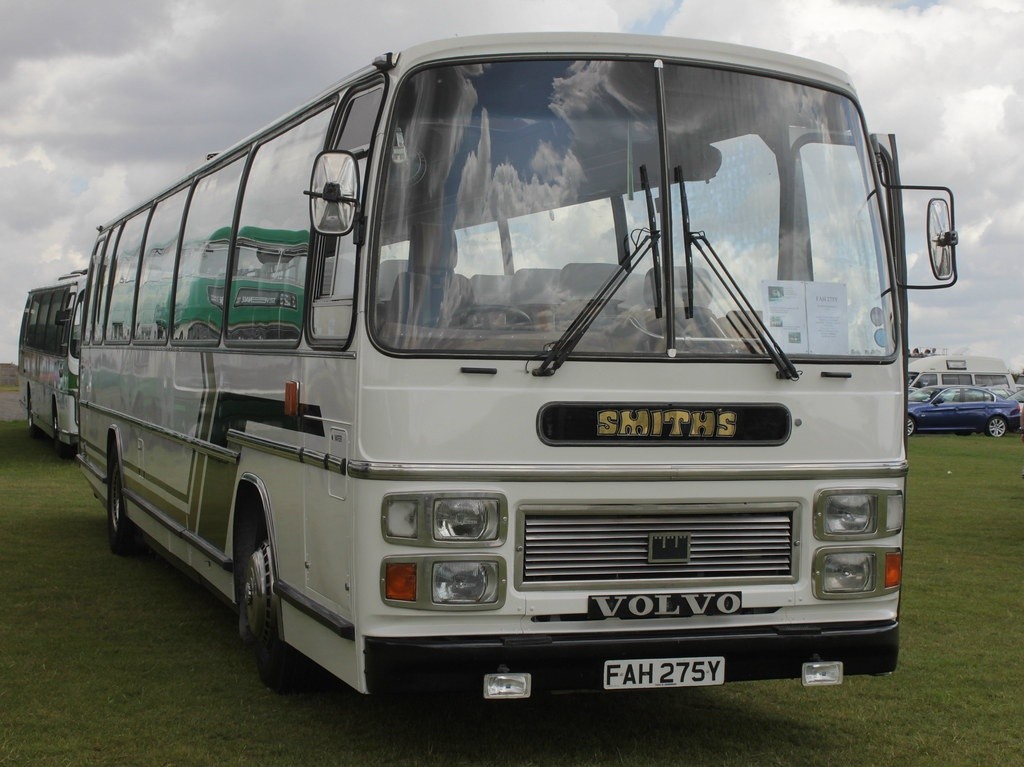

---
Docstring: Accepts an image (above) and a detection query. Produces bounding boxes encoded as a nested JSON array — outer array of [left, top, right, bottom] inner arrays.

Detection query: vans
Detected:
[[907, 355, 1017, 393]]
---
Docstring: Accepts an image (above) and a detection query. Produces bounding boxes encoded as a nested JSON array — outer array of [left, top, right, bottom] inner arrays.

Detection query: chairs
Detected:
[[953, 392, 960, 402], [378, 219, 713, 322]]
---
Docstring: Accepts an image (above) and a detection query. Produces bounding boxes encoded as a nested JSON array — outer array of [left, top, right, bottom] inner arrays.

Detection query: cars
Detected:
[[940, 380, 1024, 431], [908, 385, 1021, 439], [946, 387, 1024, 400]]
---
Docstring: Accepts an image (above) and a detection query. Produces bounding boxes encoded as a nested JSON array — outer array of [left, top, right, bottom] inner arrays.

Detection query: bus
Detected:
[[74, 31, 959, 699], [17, 269, 88, 459], [85, 225, 308, 340]]
